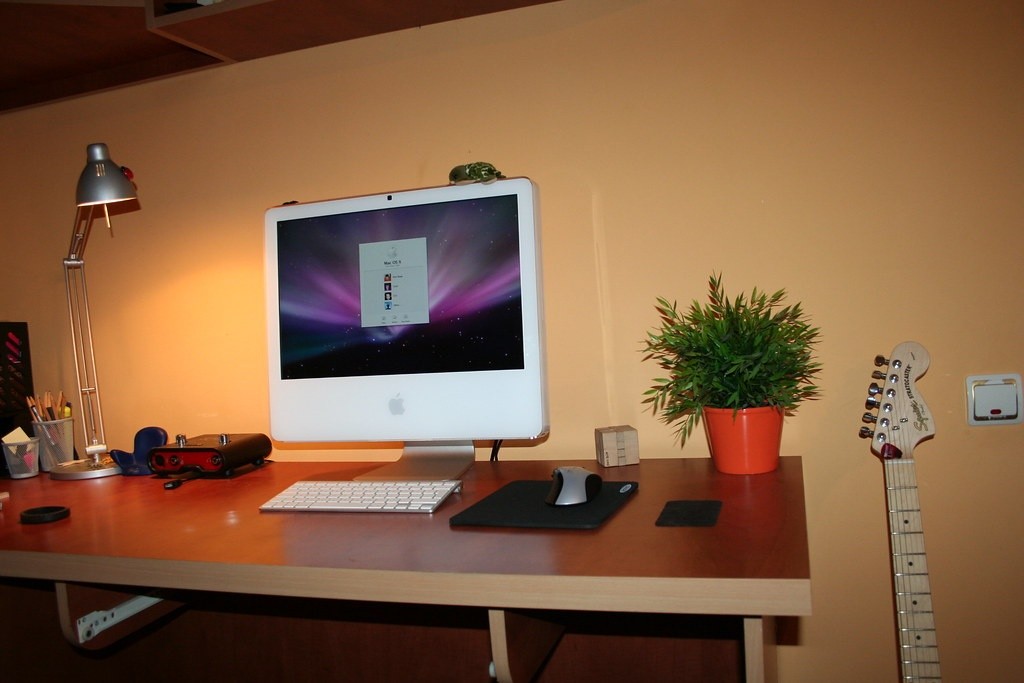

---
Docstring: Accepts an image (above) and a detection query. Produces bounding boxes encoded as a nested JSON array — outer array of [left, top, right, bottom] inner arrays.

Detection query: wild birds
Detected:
[[449, 162, 506, 184]]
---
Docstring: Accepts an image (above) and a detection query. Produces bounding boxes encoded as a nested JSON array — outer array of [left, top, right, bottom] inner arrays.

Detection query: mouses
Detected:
[[543, 463, 602, 507]]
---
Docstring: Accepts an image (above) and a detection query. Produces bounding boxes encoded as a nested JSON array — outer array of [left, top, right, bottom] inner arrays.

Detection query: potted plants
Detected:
[[640, 266, 825, 476]]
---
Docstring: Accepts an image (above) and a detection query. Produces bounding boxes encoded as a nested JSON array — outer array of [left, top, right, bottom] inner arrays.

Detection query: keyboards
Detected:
[[258, 479, 463, 515]]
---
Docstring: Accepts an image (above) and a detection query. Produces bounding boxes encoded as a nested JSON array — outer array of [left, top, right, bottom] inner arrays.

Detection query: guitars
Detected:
[[857, 341, 940, 683]]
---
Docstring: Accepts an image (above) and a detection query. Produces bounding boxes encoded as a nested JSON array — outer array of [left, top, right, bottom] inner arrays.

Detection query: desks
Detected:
[[0, 455, 814, 683]]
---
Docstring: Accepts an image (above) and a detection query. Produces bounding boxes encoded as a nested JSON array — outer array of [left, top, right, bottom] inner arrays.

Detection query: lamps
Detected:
[[48, 142, 138, 479]]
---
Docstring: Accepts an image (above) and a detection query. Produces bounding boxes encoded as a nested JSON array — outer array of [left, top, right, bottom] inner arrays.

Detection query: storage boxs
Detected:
[[0, 322, 35, 479]]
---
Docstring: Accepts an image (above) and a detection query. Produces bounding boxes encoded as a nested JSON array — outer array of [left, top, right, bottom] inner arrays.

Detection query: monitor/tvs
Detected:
[[267, 175, 550, 481]]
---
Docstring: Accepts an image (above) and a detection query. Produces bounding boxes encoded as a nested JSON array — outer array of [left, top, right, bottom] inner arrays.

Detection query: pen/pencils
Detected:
[[27, 388, 81, 473]]
